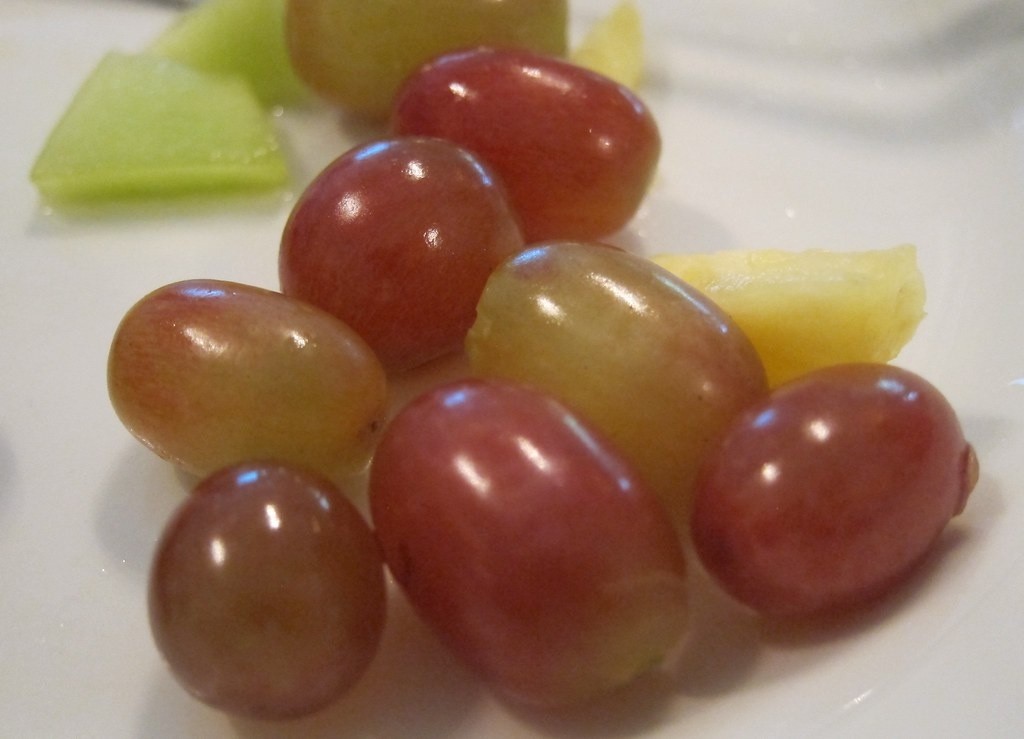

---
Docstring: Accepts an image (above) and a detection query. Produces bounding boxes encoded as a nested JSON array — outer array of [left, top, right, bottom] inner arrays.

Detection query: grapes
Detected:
[[104, 0, 979, 720]]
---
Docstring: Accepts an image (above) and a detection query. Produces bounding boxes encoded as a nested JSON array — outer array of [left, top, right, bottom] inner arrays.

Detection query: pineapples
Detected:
[[639, 246, 924, 387], [573, 5, 641, 78]]
